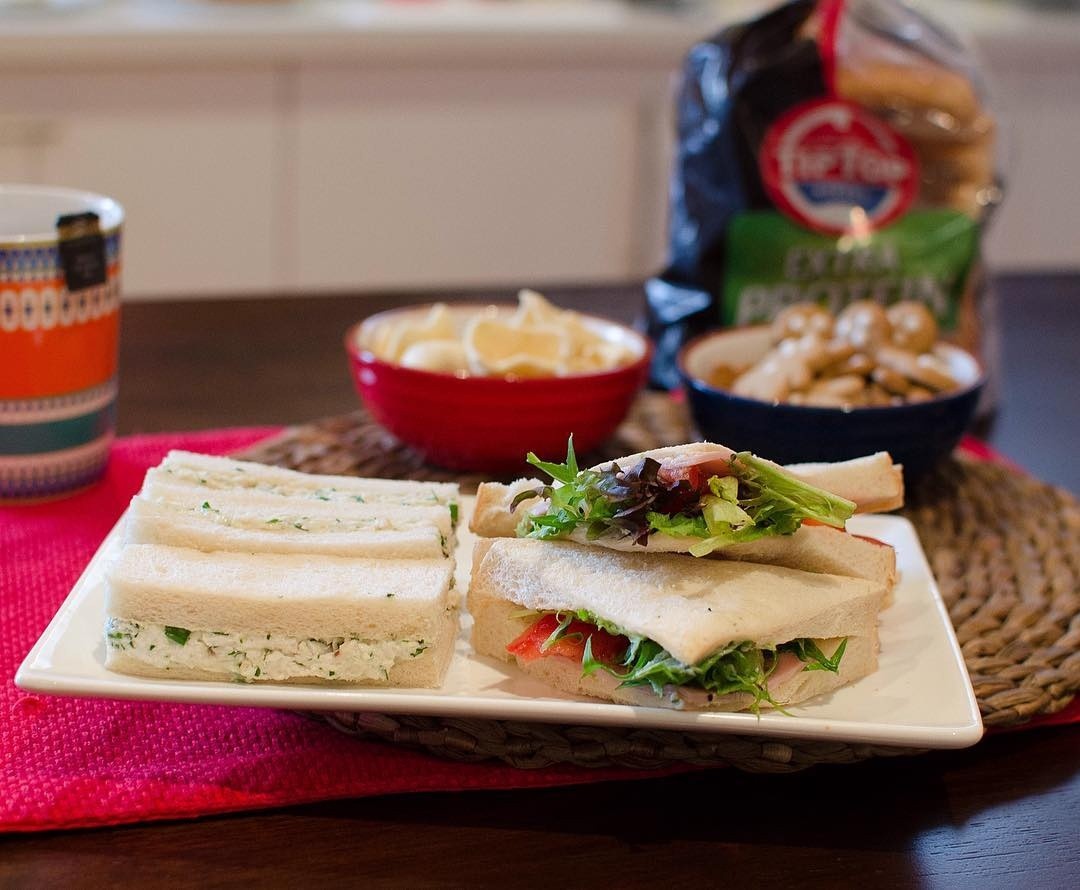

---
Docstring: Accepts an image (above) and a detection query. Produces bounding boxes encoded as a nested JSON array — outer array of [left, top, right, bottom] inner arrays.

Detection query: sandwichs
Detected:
[[104, 440, 907, 714]]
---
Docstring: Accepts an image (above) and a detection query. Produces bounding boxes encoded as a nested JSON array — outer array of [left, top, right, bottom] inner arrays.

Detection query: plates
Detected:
[[14, 510, 983, 749]]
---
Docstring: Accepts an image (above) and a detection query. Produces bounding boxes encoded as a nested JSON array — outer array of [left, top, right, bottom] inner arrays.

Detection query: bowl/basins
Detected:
[[673, 323, 993, 488], [345, 303, 653, 475]]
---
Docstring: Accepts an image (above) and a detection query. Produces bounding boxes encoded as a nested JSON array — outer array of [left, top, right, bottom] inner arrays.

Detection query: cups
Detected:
[[0, 187, 129, 505]]
